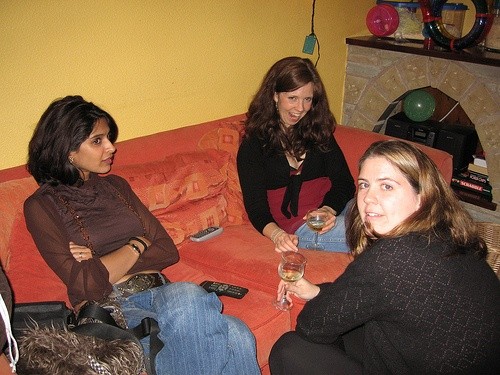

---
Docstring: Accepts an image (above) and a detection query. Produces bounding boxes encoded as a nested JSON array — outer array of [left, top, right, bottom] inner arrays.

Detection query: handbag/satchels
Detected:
[[11, 301, 74, 348]]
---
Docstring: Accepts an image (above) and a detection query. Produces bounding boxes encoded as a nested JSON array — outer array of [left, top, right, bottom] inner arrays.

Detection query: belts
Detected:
[[115, 273, 171, 300]]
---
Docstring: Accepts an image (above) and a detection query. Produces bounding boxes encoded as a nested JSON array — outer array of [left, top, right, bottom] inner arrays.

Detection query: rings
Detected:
[[79, 252, 83, 258]]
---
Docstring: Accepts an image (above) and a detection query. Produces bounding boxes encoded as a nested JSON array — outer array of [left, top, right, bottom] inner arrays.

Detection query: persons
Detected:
[[237, 56, 351, 254], [22, 94, 263, 375], [268, 138, 500, 375]]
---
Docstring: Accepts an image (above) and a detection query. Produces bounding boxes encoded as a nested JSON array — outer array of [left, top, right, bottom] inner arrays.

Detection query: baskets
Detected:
[[474, 222, 500, 279]]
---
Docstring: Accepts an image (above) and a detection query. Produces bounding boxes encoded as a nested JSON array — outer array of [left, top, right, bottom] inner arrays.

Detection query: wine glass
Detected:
[[306, 209, 328, 250], [273, 251, 307, 311]]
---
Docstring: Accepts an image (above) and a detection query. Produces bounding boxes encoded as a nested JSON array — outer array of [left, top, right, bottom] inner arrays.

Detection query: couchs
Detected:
[[0, 107, 452, 375]]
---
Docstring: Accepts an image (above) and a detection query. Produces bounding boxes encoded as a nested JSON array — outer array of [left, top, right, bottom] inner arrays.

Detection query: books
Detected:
[[451, 150, 491, 198]]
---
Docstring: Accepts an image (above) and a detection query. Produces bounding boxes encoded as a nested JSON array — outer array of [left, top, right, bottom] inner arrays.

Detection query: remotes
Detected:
[[200, 281, 249, 299], [190, 226, 223, 242]]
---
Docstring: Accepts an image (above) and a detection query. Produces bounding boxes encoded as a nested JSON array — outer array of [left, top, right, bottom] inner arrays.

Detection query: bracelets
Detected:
[[125, 242, 141, 257], [129, 237, 148, 251], [270, 228, 284, 242]]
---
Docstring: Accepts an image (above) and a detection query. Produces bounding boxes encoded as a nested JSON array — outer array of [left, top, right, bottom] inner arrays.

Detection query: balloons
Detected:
[[366, 3, 400, 38], [404, 90, 436, 123]]
